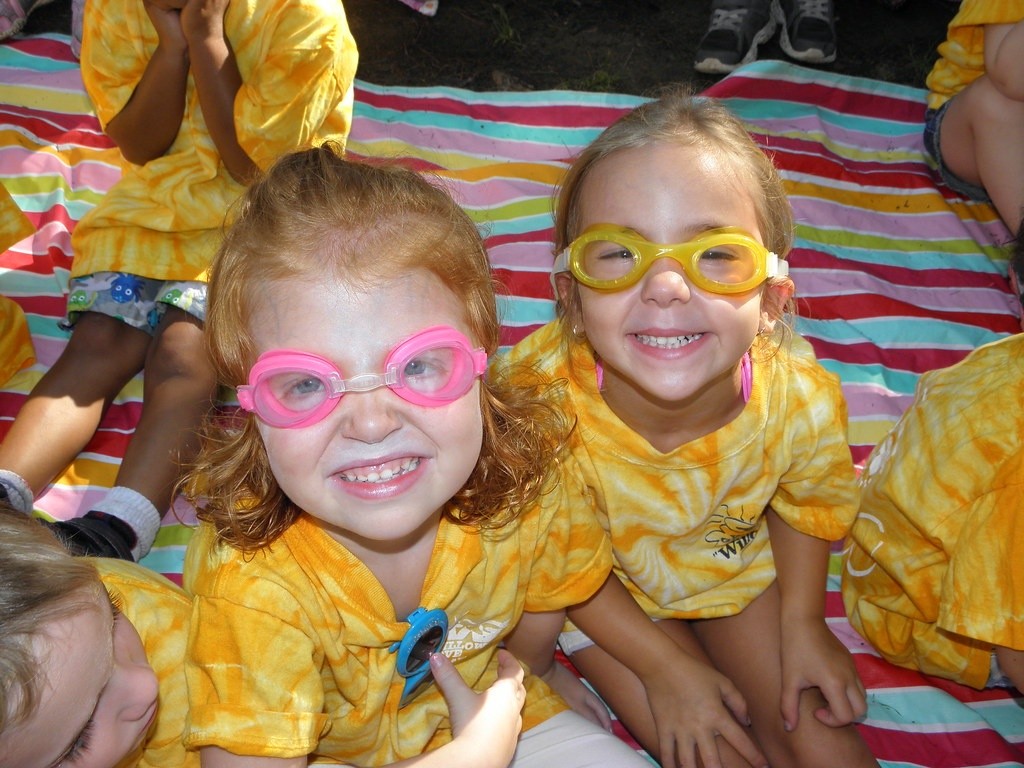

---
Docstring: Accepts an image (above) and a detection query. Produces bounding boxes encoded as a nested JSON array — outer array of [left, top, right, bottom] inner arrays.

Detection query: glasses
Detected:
[[549, 221, 790, 303], [389, 606, 451, 703], [237, 327, 487, 429]]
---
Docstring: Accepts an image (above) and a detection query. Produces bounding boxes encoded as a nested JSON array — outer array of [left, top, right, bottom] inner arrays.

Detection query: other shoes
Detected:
[[1, 484, 138, 565]]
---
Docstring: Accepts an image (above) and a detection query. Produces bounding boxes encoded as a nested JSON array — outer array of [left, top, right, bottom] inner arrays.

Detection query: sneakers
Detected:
[[693, 0, 840, 73]]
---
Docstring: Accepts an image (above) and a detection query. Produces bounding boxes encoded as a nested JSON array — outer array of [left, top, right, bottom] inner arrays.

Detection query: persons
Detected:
[[839, 215, 1024, 698], [185, 140, 659, 768], [489, 91, 883, 768], [0, 0, 359, 563], [0, 505, 201, 768], [687, 0, 842, 74], [921, 1, 1024, 240]]
